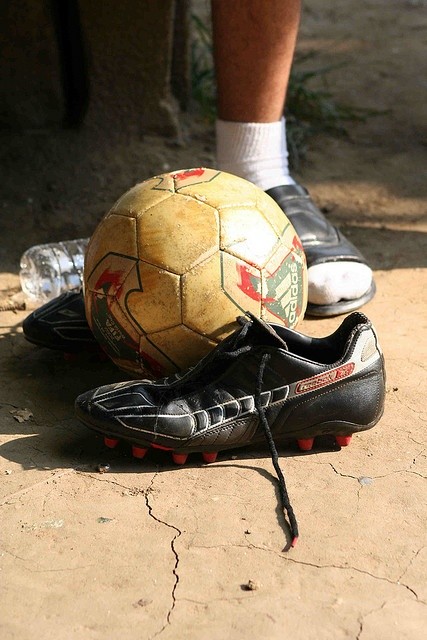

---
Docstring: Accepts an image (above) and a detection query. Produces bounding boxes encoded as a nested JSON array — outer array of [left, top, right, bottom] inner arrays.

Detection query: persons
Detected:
[[210, 0, 376, 319]]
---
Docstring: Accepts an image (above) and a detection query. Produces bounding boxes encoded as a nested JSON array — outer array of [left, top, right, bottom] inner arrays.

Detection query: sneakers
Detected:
[[21, 280, 97, 349], [74, 311, 387, 550]]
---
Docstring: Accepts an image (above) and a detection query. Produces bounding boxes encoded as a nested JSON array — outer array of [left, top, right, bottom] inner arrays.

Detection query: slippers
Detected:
[[263, 180, 377, 319]]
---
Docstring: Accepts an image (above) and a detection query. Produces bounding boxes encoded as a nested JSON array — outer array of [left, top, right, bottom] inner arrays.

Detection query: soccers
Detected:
[[83, 166, 310, 379]]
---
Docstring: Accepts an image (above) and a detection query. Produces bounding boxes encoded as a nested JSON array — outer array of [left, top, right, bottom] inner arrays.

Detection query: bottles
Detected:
[[22, 239, 106, 304]]
